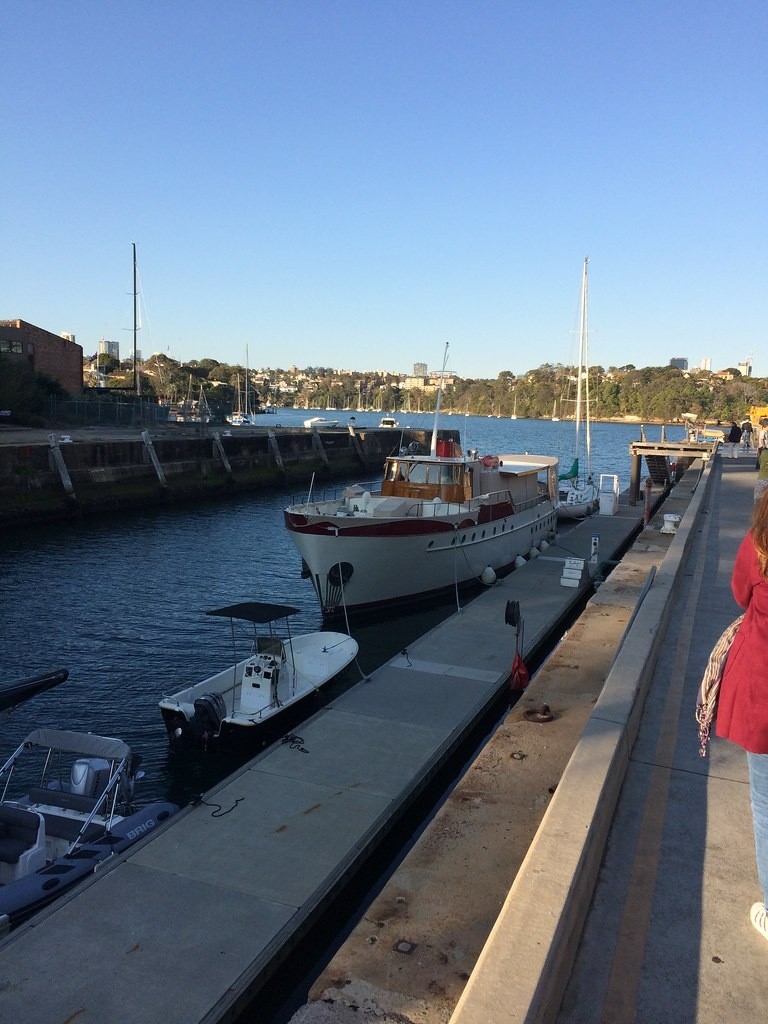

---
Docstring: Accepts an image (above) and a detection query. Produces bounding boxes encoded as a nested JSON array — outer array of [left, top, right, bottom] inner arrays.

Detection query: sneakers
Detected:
[[750, 902, 768, 941]]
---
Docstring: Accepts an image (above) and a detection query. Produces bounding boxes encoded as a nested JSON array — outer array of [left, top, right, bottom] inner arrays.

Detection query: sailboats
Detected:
[[558, 256, 601, 518], [226, 343, 258, 427]]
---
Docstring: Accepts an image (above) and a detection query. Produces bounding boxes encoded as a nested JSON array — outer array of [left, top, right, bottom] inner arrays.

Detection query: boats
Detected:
[[0, 730, 180, 939], [158, 601, 360, 762], [379, 418, 398, 428], [304, 417, 339, 428], [284, 341, 559, 615]]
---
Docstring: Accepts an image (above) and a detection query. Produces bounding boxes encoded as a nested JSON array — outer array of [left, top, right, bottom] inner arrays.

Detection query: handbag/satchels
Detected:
[[694, 614, 747, 758]]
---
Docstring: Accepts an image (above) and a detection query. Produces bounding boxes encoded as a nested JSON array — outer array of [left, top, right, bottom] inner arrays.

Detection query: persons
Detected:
[[714, 487, 768, 939], [727, 418, 768, 506]]
